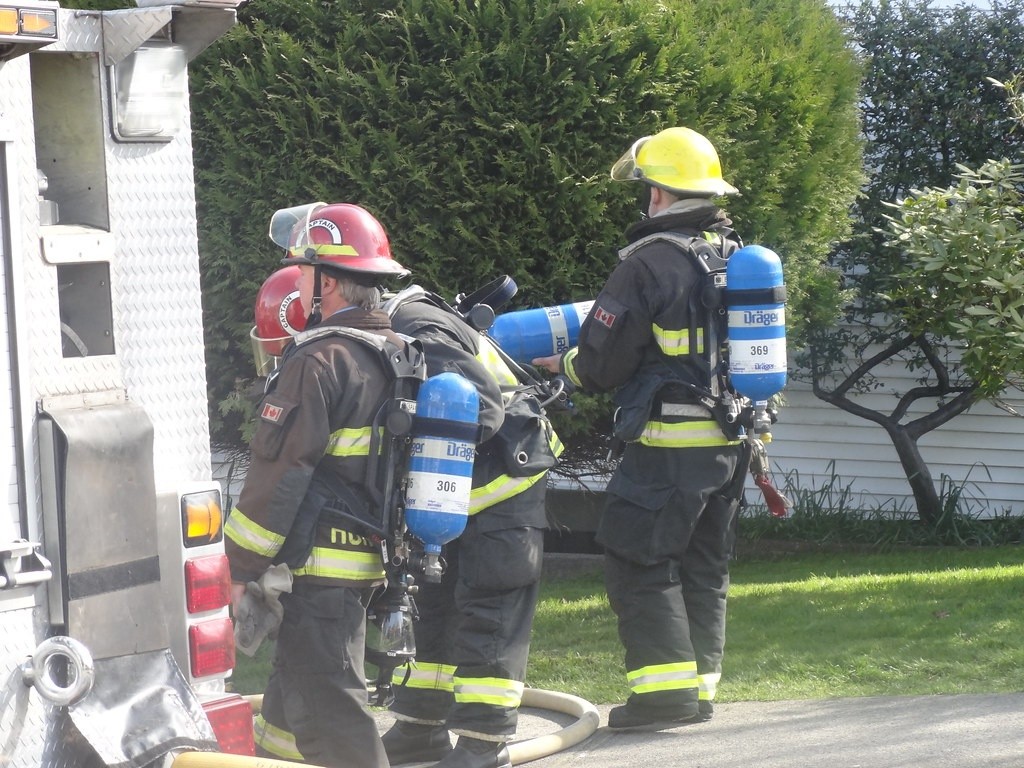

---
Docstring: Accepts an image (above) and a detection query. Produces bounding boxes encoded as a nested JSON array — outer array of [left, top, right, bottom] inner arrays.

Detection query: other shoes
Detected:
[[608, 700, 714, 731]]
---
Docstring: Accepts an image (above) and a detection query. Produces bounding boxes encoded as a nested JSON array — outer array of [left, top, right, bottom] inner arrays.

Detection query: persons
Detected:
[[532, 127, 777, 731], [225, 201, 428, 768], [250, 265, 566, 767]]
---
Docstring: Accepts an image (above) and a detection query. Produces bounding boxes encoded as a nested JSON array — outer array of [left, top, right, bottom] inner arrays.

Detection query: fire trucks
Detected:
[[0, 0, 259, 768]]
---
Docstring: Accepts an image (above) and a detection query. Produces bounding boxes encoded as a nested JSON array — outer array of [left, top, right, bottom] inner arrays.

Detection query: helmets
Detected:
[[250, 265, 307, 376], [268, 202, 407, 274], [611, 127, 739, 194]]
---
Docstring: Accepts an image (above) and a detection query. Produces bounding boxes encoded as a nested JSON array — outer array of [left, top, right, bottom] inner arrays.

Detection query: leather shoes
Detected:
[[378, 726, 453, 766], [426, 742, 512, 768]]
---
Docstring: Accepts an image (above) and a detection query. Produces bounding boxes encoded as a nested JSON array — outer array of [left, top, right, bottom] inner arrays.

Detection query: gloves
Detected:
[[229, 560, 294, 651]]
